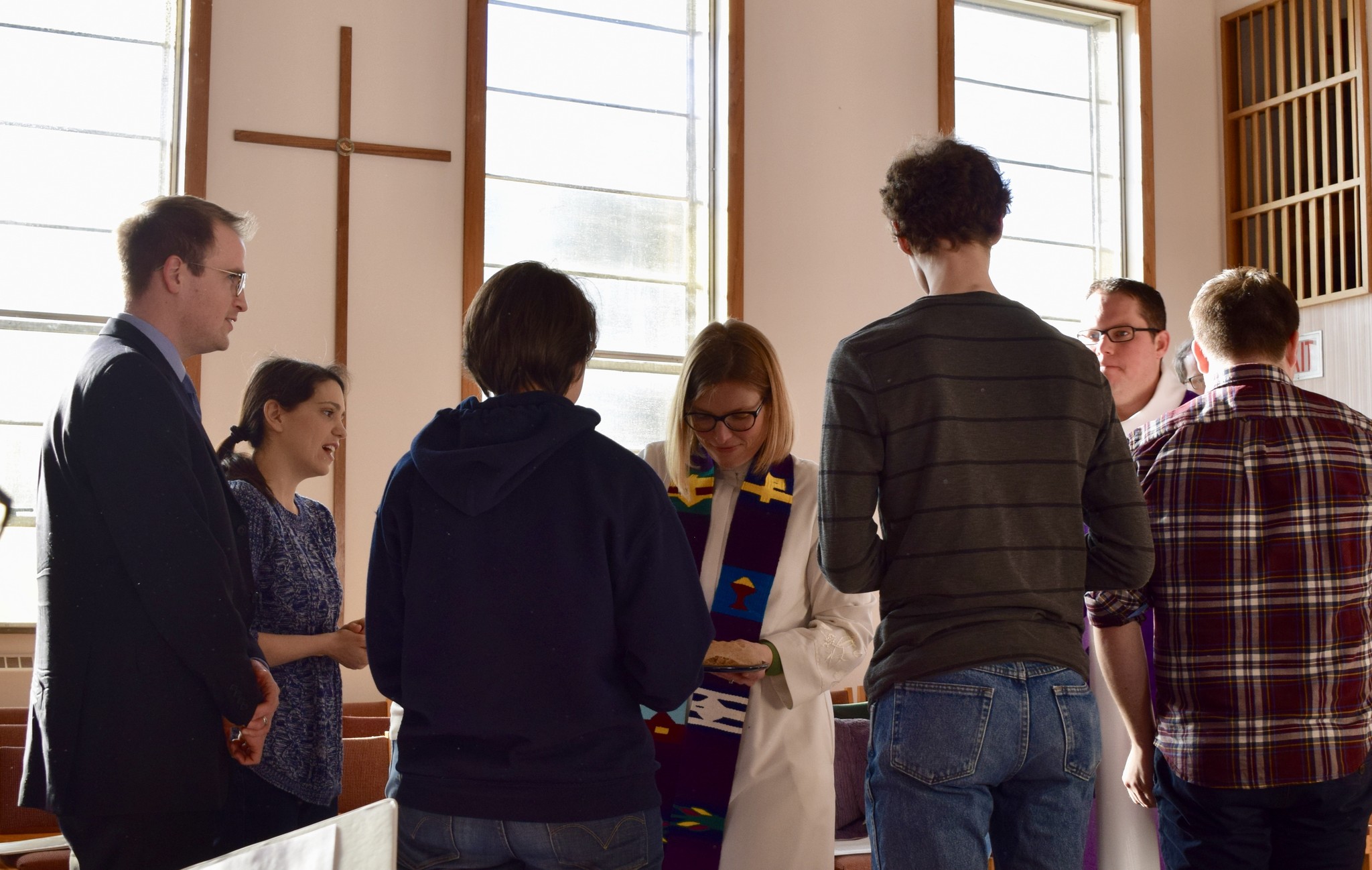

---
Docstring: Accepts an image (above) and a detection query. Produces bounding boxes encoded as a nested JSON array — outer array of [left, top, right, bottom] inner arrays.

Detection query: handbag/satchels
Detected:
[[834, 718, 871, 842]]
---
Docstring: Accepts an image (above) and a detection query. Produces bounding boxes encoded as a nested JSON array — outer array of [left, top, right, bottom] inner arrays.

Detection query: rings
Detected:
[[263, 716, 267, 725]]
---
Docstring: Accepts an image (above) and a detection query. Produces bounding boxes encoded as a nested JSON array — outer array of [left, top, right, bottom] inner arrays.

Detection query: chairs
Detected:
[[0, 680, 881, 870]]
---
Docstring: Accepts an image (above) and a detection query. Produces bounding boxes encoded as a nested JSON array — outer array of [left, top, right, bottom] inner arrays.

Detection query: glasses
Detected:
[[155, 261, 247, 297], [684, 398, 766, 432], [1077, 327, 1160, 345], [1184, 374, 1205, 390]]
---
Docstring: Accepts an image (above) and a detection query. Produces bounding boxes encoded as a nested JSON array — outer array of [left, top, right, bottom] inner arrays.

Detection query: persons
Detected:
[[384, 702, 404, 800], [1174, 336, 1206, 396], [816, 140, 1156, 870], [215, 358, 370, 837], [1074, 275, 1202, 870], [1081, 269, 1372, 870], [367, 260, 714, 870], [630, 320, 880, 870], [15, 194, 280, 870]]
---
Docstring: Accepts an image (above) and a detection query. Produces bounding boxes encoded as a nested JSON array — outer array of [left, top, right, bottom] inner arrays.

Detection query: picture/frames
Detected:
[[1292, 330, 1324, 382]]
